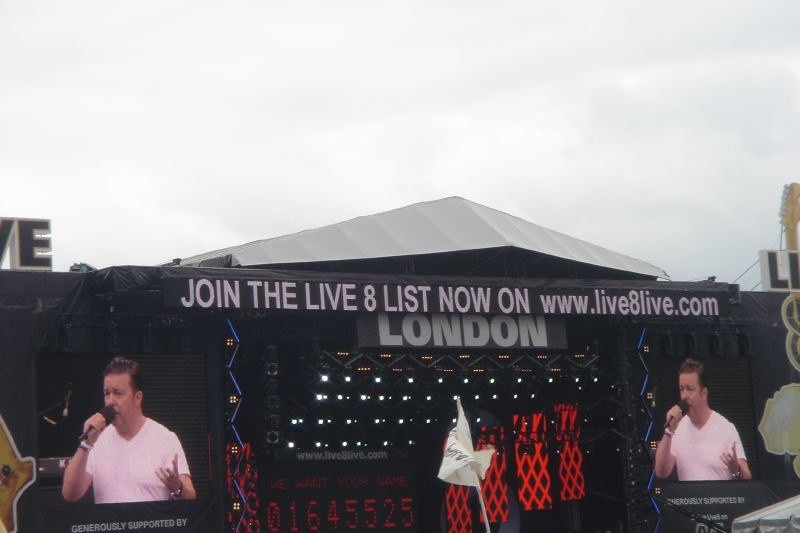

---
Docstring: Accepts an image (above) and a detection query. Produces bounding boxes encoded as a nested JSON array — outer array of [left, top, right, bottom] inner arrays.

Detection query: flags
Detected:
[[436, 400, 496, 489]]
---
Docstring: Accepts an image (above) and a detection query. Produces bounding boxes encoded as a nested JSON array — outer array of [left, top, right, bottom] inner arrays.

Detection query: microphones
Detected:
[[76, 407, 115, 441], [663, 400, 689, 428]]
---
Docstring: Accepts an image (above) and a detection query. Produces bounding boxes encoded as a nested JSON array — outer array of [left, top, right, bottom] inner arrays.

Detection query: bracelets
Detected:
[[78, 441, 94, 453], [171, 481, 184, 497], [664, 428, 675, 437], [731, 468, 743, 479]]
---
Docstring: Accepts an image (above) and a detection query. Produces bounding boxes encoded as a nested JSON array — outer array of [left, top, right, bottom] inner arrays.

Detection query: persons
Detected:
[[653, 359, 752, 482], [62, 356, 197, 504]]
